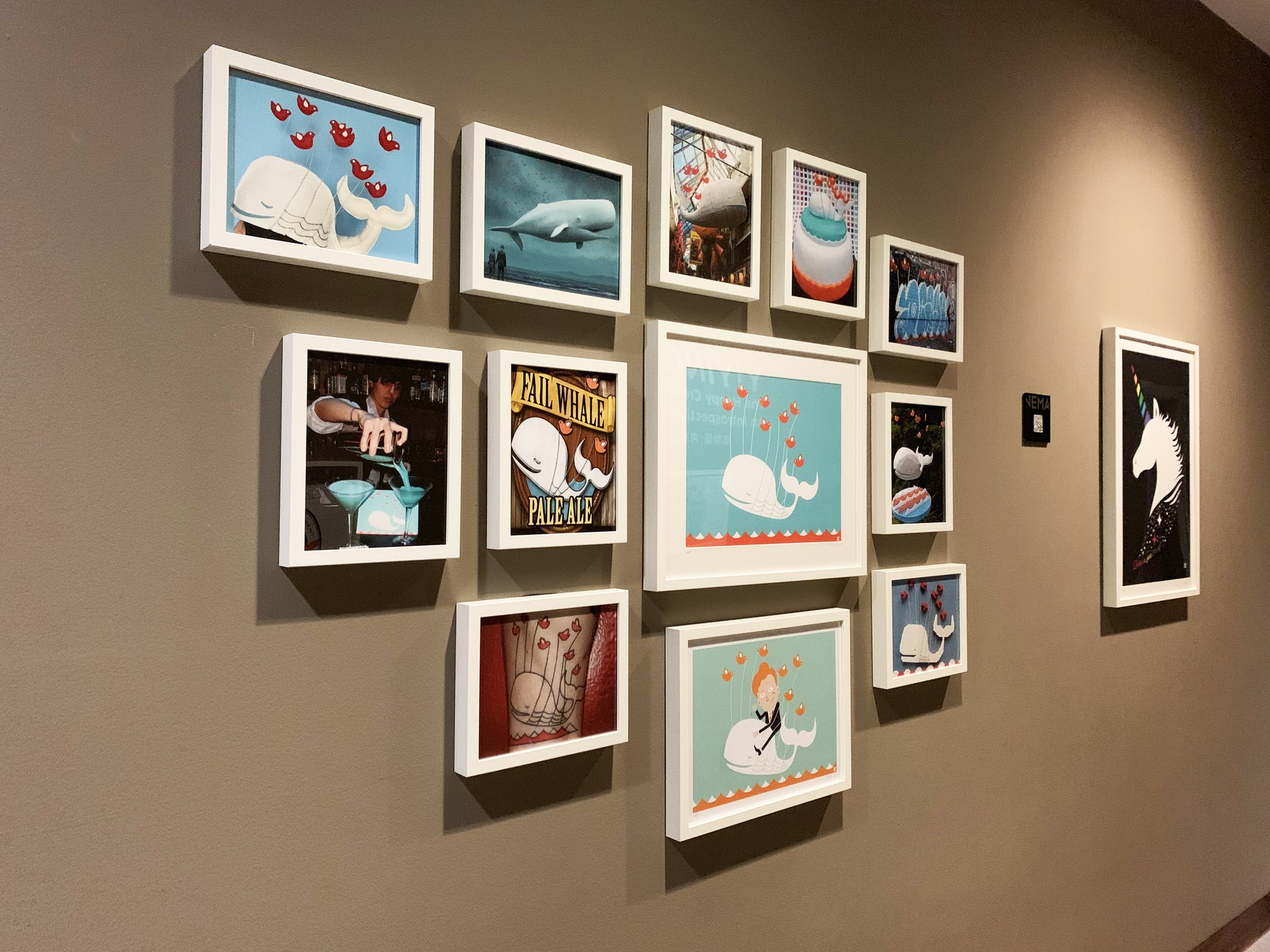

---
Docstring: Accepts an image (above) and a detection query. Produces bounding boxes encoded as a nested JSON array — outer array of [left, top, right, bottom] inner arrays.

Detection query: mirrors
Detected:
[[198, 44, 436, 288]]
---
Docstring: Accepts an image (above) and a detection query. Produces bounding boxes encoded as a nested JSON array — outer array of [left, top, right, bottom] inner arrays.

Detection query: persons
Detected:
[[487, 248, 497, 278], [495, 244, 508, 282], [308, 363, 413, 485]]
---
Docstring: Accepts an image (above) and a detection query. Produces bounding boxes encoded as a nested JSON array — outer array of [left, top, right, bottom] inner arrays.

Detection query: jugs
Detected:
[[333, 428, 405, 471]]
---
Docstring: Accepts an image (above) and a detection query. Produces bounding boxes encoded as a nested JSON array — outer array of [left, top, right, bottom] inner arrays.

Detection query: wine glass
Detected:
[[389, 475, 432, 545], [324, 475, 376, 549]]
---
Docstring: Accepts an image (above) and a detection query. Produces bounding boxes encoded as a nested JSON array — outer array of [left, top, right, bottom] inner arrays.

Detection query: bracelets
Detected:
[[351, 408, 363, 423]]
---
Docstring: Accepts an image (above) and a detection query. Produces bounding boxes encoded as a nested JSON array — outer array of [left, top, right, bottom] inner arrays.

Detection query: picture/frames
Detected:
[[870, 563, 968, 691], [646, 104, 763, 305], [770, 147, 868, 322], [454, 587, 628, 777], [870, 392, 954, 535], [486, 348, 628, 550], [279, 332, 463, 569], [643, 320, 868, 592], [1101, 326, 1201, 608], [663, 607, 853, 843], [460, 120, 632, 320], [867, 233, 965, 364]]
